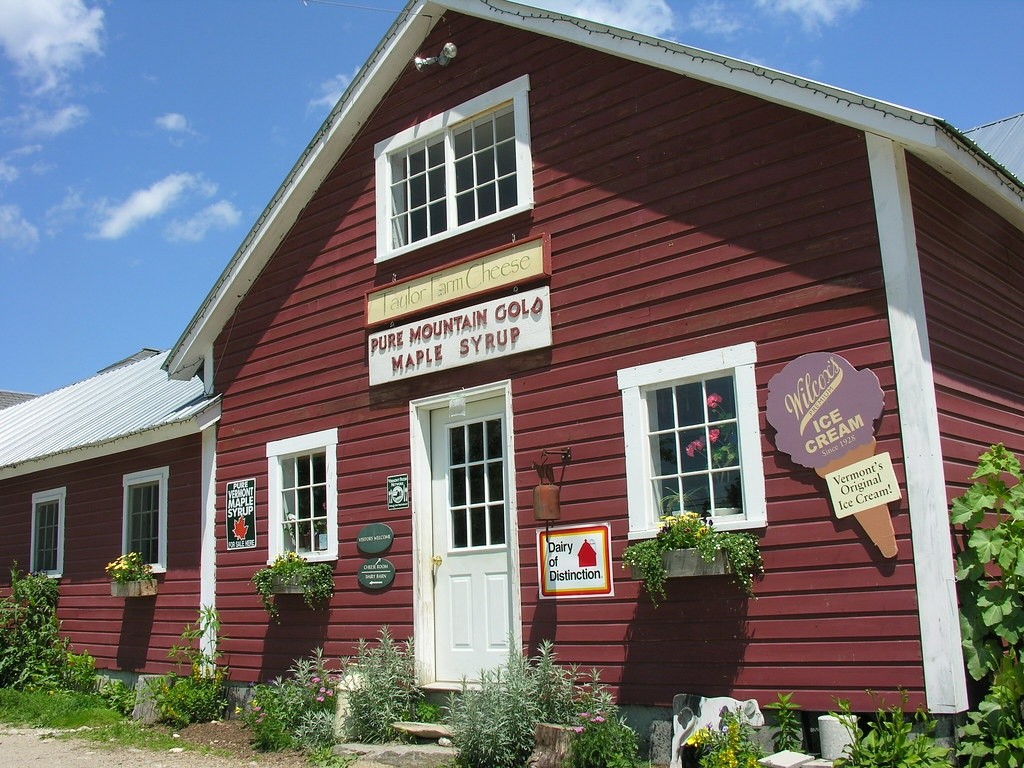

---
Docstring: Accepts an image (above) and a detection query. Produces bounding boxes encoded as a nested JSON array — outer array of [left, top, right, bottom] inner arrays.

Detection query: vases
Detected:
[[270, 575, 315, 594], [110, 579, 158, 597], [318, 533, 328, 550], [707, 507, 742, 516], [298, 534, 311, 547], [630, 546, 733, 581]]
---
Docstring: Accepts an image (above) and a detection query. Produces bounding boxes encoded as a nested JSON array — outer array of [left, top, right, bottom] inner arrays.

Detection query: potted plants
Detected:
[[658, 479, 705, 515]]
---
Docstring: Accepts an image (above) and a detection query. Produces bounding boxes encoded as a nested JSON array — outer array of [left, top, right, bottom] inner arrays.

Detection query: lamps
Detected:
[[531, 447, 572, 542]]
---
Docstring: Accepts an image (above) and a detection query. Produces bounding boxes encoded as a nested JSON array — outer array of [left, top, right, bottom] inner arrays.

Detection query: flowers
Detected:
[[621, 511, 765, 609], [283, 512, 311, 536], [314, 503, 327, 534], [105, 551, 154, 587], [246, 548, 336, 628], [410, 42, 458, 73], [685, 393, 742, 508]]
